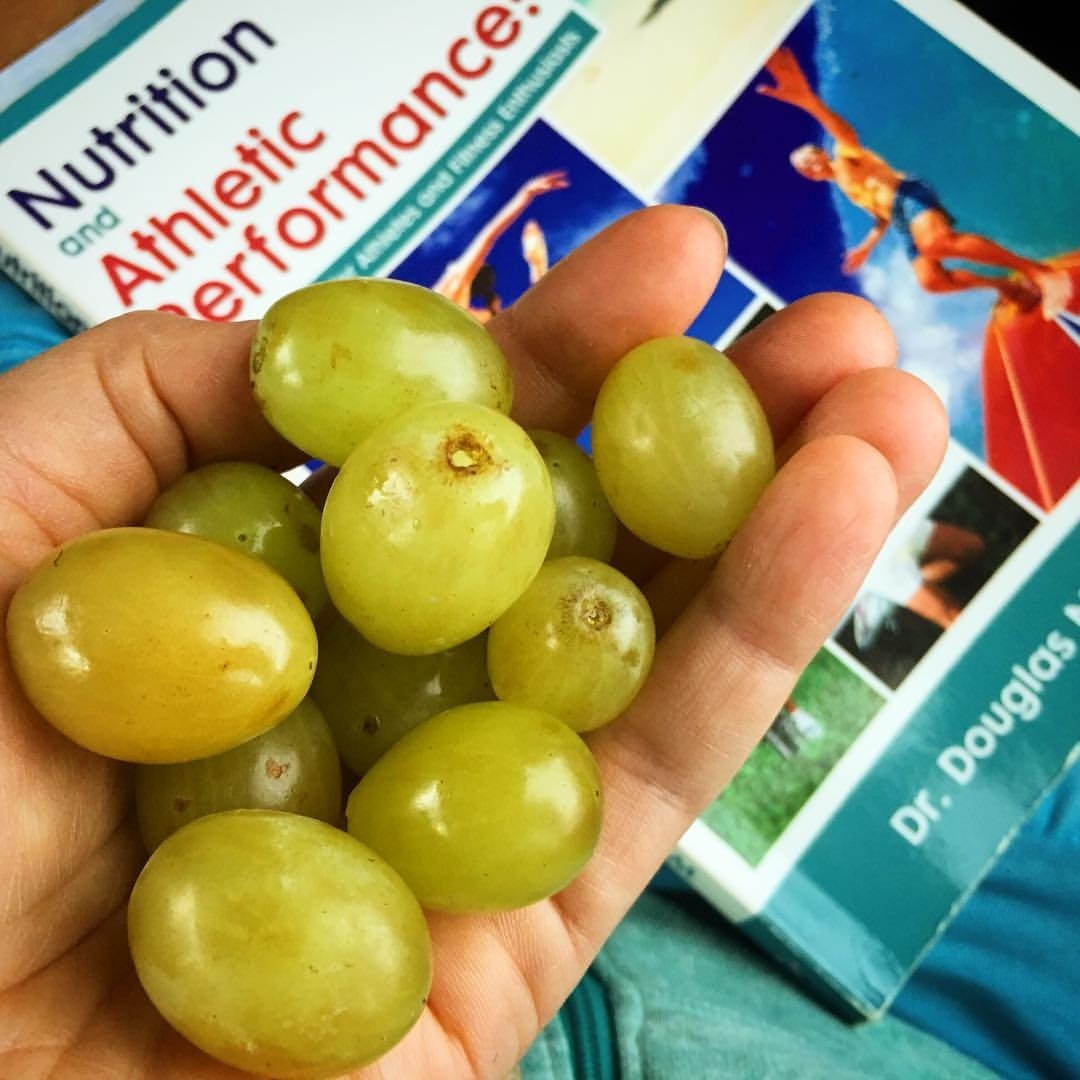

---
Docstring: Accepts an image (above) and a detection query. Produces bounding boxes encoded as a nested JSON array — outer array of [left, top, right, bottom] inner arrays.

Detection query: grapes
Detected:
[[0, 277, 770, 1080]]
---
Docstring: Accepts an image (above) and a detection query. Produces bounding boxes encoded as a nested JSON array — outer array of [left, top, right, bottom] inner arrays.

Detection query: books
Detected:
[[2, 0, 1080, 1025]]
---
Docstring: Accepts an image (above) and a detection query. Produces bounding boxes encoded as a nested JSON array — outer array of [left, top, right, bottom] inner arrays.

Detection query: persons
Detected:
[[1, 205, 948, 1080], [435, 174, 568, 322], [758, 51, 1071, 321], [871, 523, 982, 626]]
